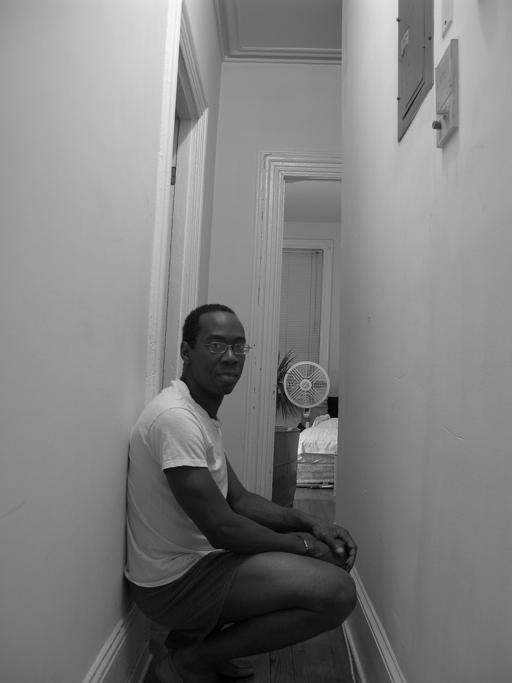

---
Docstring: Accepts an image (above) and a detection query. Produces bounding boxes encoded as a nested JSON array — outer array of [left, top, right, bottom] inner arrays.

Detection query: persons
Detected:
[[123, 304, 357, 683]]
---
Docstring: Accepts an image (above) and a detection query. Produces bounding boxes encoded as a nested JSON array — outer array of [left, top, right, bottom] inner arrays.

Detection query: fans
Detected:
[[283, 361, 330, 428]]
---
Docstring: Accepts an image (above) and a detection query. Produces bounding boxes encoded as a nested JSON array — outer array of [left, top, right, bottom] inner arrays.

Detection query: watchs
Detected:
[[296, 531, 312, 556]]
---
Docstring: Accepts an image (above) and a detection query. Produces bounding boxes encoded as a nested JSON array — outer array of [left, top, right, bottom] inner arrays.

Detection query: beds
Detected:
[[296, 397, 338, 487]]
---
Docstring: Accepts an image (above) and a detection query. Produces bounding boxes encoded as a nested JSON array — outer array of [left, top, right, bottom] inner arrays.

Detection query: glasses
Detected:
[[196, 342, 250, 355]]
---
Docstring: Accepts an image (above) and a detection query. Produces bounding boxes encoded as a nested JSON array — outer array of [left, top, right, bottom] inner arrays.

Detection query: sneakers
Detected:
[[157, 630, 253, 683]]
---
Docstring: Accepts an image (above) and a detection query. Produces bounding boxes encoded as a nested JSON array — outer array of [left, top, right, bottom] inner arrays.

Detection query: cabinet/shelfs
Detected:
[[397, 0, 434, 141]]
